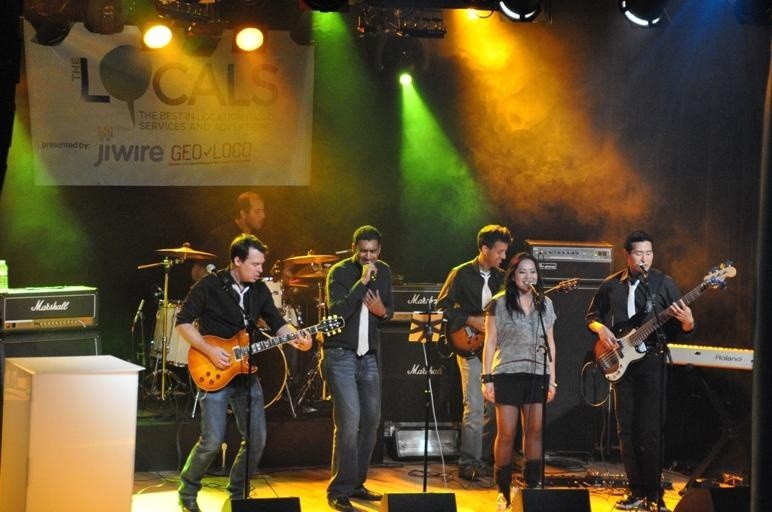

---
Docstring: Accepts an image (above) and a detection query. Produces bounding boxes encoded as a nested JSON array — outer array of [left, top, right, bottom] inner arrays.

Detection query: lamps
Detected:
[[134, 11, 172, 49], [233, 15, 264, 52], [620, 0, 666, 26], [83, 0, 124, 36], [21, 0, 70, 46], [499, 0, 542, 22]]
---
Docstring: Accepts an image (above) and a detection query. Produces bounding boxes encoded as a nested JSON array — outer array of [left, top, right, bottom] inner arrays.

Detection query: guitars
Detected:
[[445, 276, 581, 359], [187, 314, 345, 393], [595, 259, 736, 383]]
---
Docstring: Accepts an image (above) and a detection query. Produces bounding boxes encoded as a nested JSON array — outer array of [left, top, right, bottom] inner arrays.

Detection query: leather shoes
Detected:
[[353, 485, 382, 501], [329, 495, 353, 512], [180, 500, 198, 512]]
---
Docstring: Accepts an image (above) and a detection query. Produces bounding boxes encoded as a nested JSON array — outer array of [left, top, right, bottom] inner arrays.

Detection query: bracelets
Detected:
[[548, 380, 558, 388], [380, 314, 388, 320], [480, 373, 494, 383]]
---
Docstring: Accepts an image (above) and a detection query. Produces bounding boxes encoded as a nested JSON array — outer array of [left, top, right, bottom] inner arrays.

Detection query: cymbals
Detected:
[[137, 261, 181, 269], [283, 251, 340, 266], [156, 241, 216, 264], [298, 270, 329, 281], [285, 282, 311, 291]]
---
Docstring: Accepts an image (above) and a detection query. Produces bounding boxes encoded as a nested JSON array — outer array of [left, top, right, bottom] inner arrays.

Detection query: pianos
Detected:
[[609, 341, 753, 370]]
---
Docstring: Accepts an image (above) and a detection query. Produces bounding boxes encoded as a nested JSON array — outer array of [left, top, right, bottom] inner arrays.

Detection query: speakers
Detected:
[[378, 491, 457, 512], [535, 261, 612, 457], [660, 343, 753, 484], [673, 484, 751, 512], [510, 486, 592, 512], [221, 496, 302, 512], [376, 283, 462, 457]]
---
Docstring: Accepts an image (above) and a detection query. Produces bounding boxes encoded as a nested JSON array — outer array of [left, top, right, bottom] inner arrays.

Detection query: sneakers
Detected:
[[615, 497, 644, 509]]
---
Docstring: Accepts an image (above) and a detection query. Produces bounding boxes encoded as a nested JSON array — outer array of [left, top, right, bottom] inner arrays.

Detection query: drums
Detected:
[[280, 304, 302, 327], [149, 299, 192, 368], [258, 275, 284, 310]]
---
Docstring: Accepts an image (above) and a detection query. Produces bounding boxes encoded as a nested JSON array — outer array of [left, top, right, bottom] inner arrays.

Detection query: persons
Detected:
[[189, 189, 302, 289], [318, 223, 394, 510], [434, 223, 516, 481], [583, 231, 697, 512], [175, 234, 315, 512], [481, 253, 557, 510]]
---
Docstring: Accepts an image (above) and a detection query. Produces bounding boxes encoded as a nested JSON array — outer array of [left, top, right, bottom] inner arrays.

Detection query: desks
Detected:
[[0, 353, 147, 512]]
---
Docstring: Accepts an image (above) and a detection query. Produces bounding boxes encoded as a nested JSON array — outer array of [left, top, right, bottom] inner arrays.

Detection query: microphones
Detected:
[[206, 263, 229, 286], [361, 259, 376, 285], [526, 279, 545, 301], [636, 262, 649, 279]]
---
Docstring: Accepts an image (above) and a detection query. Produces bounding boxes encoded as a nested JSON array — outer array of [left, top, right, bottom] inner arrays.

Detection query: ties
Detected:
[[357, 302, 369, 357], [480, 272, 493, 310], [627, 280, 640, 319]]
